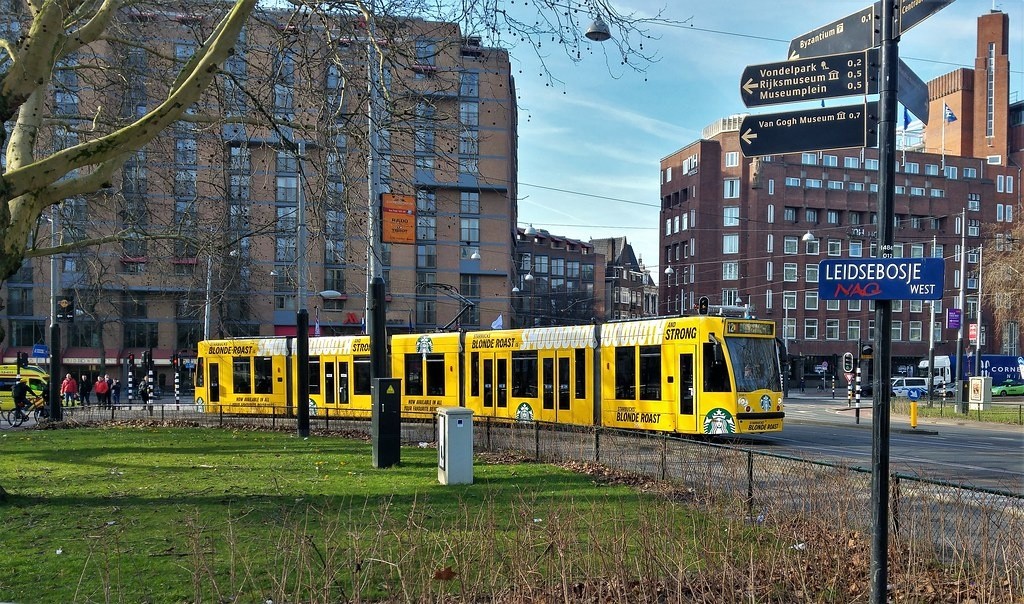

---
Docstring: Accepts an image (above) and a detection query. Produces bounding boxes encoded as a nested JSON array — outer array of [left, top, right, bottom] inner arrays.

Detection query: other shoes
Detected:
[[21, 408, 26, 414]]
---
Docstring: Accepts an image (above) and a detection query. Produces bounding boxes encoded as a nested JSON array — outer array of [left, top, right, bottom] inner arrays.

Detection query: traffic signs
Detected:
[[897, 56, 930, 126], [786, 4, 874, 62], [739, 47, 881, 108], [739, 101, 879, 158]]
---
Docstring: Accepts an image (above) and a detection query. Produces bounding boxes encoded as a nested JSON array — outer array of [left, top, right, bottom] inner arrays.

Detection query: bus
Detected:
[[191, 282, 787, 442]]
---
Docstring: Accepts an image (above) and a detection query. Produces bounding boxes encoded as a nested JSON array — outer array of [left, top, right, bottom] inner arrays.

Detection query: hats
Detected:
[[20, 379, 27, 382]]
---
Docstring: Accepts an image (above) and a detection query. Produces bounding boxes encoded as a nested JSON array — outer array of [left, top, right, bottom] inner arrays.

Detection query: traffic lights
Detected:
[[861, 344, 873, 356], [842, 351, 853, 372], [128, 353, 134, 372], [170, 355, 174, 369]]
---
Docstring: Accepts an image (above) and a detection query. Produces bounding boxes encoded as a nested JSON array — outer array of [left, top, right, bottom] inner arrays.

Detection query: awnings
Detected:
[[62, 347, 119, 366], [3, 347, 50, 365], [119, 348, 174, 366]]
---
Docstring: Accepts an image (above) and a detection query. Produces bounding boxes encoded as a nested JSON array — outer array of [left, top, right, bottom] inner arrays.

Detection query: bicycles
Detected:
[[7, 394, 50, 428], [0, 400, 17, 430]]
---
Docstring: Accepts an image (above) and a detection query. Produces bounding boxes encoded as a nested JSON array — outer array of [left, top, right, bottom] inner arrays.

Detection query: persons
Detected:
[[103, 373, 113, 409], [13, 378, 40, 419], [94, 376, 109, 410], [800, 376, 806, 392], [41, 381, 49, 405], [109, 378, 121, 410], [76, 374, 92, 410], [61, 372, 77, 406], [139, 376, 149, 410]]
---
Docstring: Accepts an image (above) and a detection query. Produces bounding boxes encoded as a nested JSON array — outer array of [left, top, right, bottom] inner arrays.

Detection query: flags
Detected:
[[944, 105, 956, 124], [491, 315, 503, 330], [903, 107, 912, 130]]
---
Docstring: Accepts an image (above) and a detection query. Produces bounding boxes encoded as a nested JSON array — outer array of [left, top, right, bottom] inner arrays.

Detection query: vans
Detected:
[[891, 377, 929, 399], [0, 364, 79, 411]]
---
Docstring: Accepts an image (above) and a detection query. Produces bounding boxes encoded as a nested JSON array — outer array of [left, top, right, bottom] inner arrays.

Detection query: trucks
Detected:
[[917, 352, 1024, 398]]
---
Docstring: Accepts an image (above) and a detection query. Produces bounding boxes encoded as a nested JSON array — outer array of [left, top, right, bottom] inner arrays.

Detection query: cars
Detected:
[[933, 382, 956, 397], [861, 382, 872, 397]]
